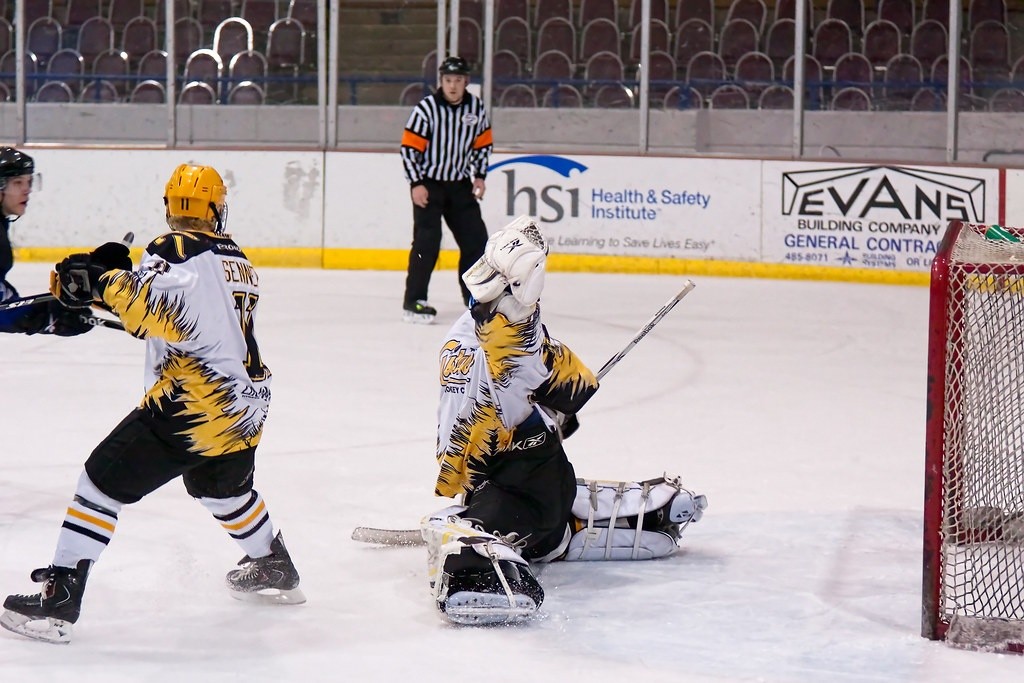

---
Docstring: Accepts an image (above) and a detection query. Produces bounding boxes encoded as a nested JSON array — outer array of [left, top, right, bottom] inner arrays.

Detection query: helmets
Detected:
[[438, 56, 472, 76], [159, 163, 232, 230], [0, 145, 41, 193]]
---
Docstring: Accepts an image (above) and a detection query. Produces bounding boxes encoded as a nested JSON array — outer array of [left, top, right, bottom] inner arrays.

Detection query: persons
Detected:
[[418, 213, 708, 625], [399, 57, 494, 324], [0, 164, 306, 643], [0, 146, 95, 337]]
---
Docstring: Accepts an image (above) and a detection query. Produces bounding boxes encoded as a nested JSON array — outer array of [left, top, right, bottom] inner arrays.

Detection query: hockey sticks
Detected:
[[351, 279, 700, 547], [0, 232, 135, 313], [80, 313, 124, 331]]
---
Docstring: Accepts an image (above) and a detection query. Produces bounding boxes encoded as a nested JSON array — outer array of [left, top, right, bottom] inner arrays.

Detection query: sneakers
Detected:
[[226, 527, 308, 607], [2, 556, 95, 649], [401, 300, 438, 325], [441, 577, 546, 628]]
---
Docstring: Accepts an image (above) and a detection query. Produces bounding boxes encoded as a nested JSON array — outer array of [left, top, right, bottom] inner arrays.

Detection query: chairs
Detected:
[[0, 0, 317, 106], [400, 0, 1024, 113]]
